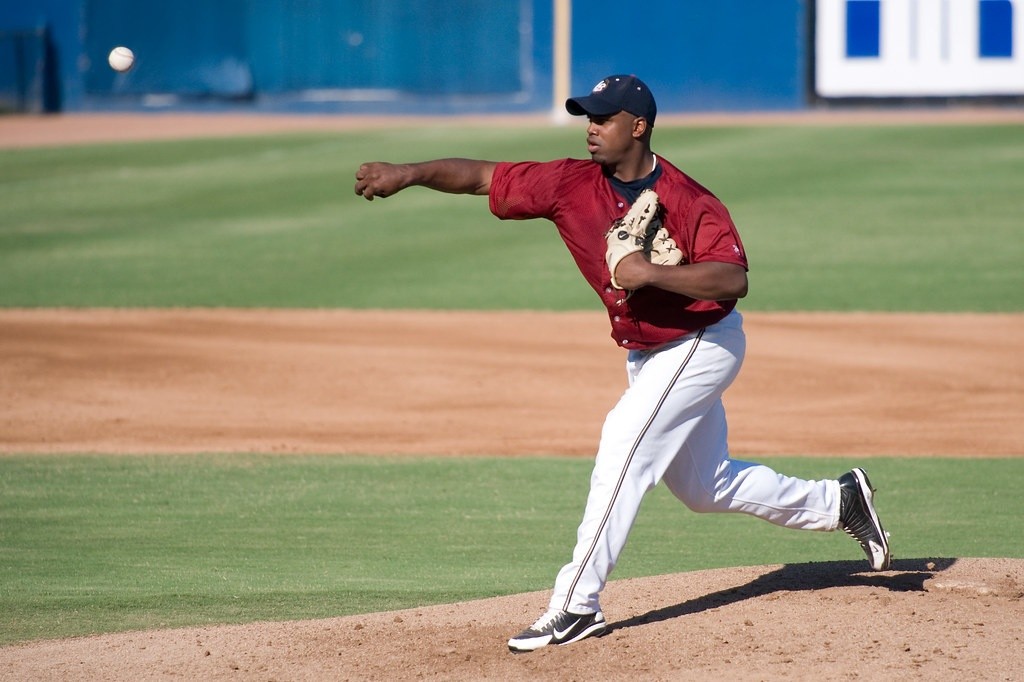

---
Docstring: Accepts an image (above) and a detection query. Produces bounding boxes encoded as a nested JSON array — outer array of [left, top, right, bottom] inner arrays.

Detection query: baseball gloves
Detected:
[[605, 188, 686, 292]]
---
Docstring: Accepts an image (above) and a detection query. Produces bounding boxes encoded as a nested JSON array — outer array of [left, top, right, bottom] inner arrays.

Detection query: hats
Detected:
[[566, 75, 657, 126]]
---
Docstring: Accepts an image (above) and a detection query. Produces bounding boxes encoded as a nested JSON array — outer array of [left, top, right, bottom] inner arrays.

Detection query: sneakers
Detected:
[[507, 607, 607, 650], [837, 467, 891, 572]]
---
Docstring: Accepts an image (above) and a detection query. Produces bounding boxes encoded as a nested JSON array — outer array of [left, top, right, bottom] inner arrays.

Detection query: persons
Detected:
[[354, 74, 893, 654]]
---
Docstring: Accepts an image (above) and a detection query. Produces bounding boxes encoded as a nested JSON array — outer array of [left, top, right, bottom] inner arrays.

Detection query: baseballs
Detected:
[[107, 46, 133, 74]]
